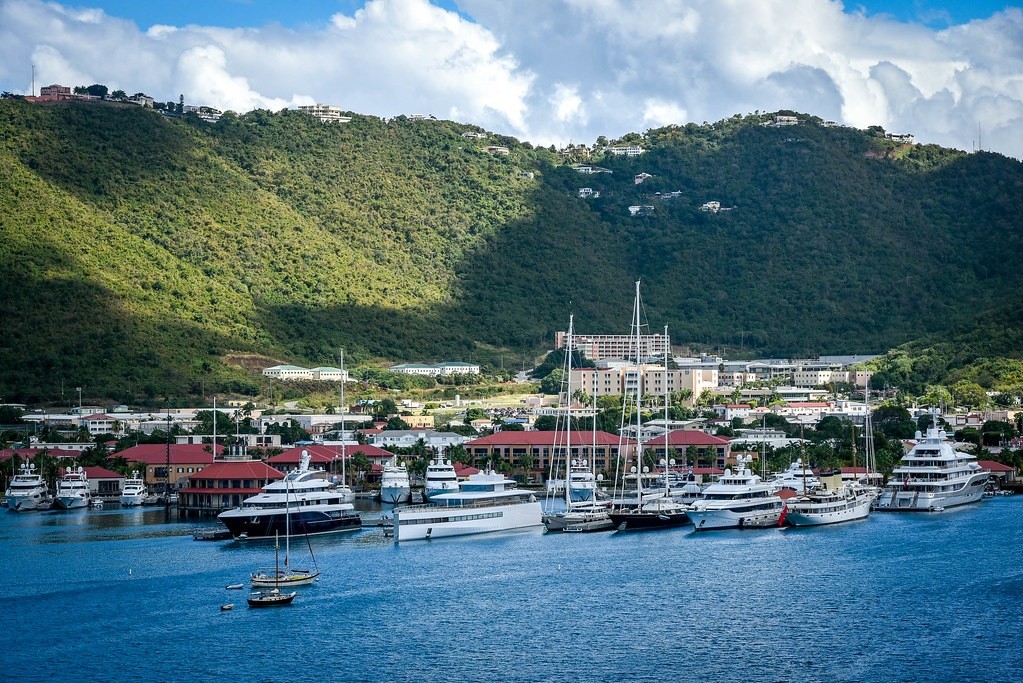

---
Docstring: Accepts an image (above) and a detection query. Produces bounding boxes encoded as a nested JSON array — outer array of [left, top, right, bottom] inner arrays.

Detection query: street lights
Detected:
[[76, 387, 81, 418]]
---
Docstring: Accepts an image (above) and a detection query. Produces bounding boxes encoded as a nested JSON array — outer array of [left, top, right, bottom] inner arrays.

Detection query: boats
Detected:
[[546, 459, 597, 494], [423, 446, 459, 502], [221, 604, 234, 611], [1, 502, 8, 507], [91, 499, 103, 507], [873, 405, 991, 510], [681, 455, 785, 531], [380, 456, 410, 502], [781, 418, 884, 525], [5, 458, 52, 511], [53, 462, 90, 508], [379, 459, 543, 541], [624, 455, 823, 505], [226, 583, 243, 589], [119, 470, 148, 506]]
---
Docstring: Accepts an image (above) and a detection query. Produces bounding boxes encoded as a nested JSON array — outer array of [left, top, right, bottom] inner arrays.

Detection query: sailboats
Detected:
[[247, 530, 296, 607], [328, 349, 356, 502], [250, 471, 321, 587], [607, 279, 694, 529], [542, 313, 627, 531], [218, 450, 362, 542]]
